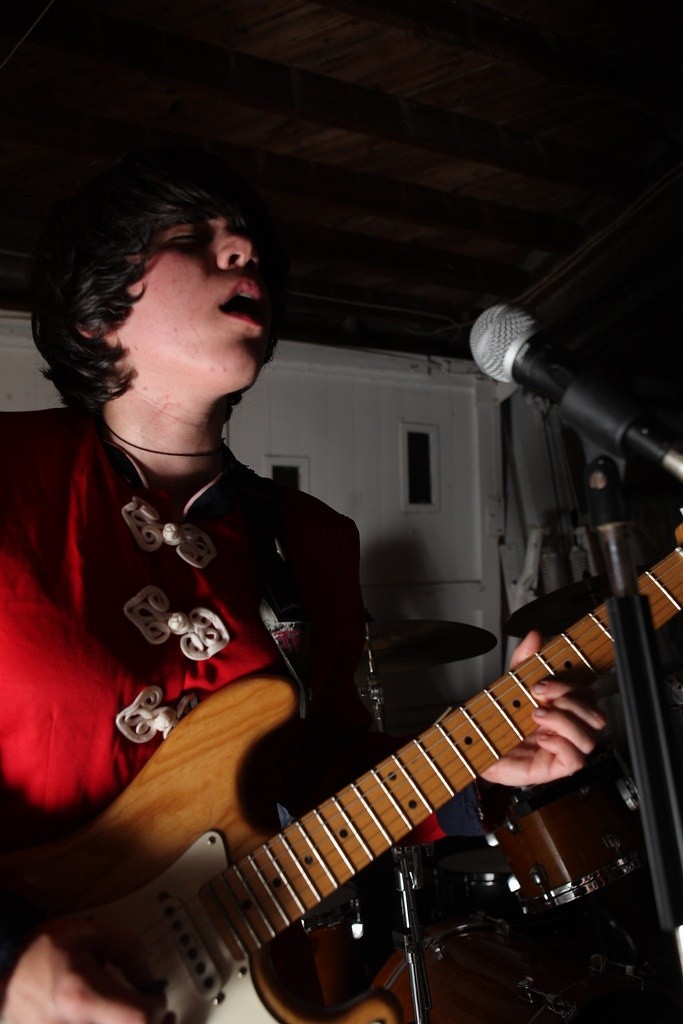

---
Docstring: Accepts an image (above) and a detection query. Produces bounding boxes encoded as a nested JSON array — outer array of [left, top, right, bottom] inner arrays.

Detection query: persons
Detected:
[[1, 172, 607, 1023]]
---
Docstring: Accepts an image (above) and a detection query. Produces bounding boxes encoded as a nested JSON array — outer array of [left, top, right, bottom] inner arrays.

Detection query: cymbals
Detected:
[[345, 617, 499, 679], [499, 565, 652, 640]]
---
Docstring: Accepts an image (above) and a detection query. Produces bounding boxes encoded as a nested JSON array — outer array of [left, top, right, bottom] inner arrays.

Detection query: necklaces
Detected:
[[106, 424, 226, 457]]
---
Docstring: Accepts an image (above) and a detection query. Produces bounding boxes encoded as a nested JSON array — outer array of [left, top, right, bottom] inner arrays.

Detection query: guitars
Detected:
[[0, 506, 683, 1024]]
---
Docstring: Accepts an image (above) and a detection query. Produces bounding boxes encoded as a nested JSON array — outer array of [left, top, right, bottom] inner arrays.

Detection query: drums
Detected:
[[476, 744, 650, 917], [430, 845, 597, 939], [368, 909, 683, 1024], [273, 880, 362, 1014]]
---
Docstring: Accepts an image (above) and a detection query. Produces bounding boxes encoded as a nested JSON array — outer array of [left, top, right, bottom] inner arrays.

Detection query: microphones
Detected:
[[469, 303, 683, 483]]
